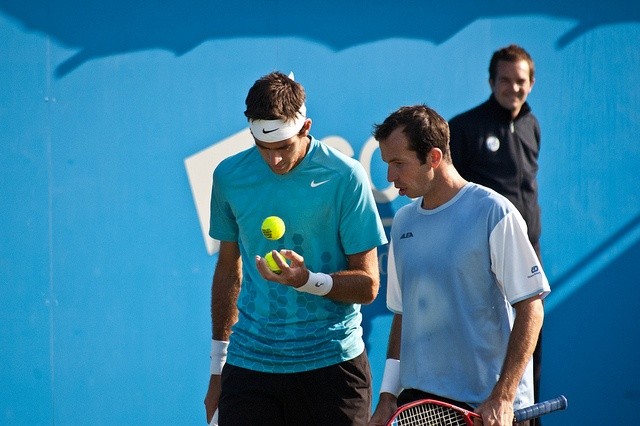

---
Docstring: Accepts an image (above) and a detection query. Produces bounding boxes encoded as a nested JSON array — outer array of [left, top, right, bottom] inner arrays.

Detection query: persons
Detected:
[[366, 104, 552, 425], [448, 45, 541, 425], [203, 72, 389, 426]]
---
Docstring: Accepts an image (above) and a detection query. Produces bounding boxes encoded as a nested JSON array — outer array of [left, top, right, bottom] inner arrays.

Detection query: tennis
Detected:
[[265, 251, 287, 274], [261, 216, 285, 240]]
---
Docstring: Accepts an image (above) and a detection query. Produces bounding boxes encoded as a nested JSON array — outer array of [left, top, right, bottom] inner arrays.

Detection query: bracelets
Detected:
[[379, 357, 405, 399], [293, 269, 334, 297], [210, 339, 229, 375]]
